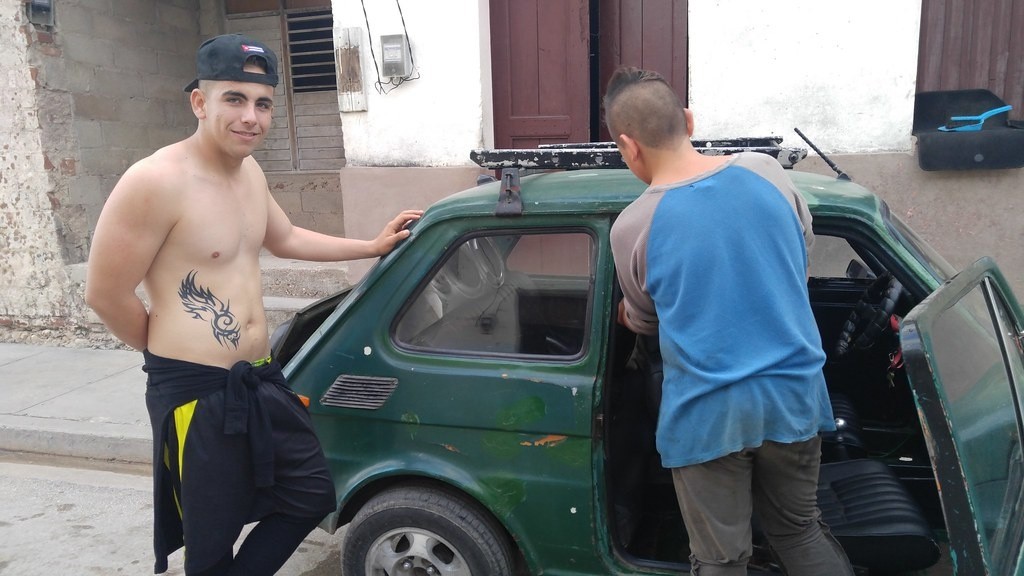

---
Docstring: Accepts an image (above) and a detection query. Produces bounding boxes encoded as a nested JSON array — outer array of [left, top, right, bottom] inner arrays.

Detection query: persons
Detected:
[[84, 33, 424, 576], [605, 66, 856, 576]]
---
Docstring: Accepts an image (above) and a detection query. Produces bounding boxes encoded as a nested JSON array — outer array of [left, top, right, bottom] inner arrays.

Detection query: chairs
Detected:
[[751, 459, 942, 576], [822, 393, 868, 464]]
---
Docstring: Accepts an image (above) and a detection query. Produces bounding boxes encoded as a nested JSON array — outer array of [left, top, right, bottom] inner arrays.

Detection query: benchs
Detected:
[[409, 271, 571, 354]]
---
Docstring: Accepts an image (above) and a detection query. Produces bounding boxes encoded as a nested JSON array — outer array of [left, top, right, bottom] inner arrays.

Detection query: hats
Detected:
[[183, 32, 278, 93]]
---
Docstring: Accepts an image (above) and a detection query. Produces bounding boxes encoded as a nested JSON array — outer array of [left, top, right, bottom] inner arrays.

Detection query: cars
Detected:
[[248, 137, 1022, 576], [914, 89, 1024, 171]]
